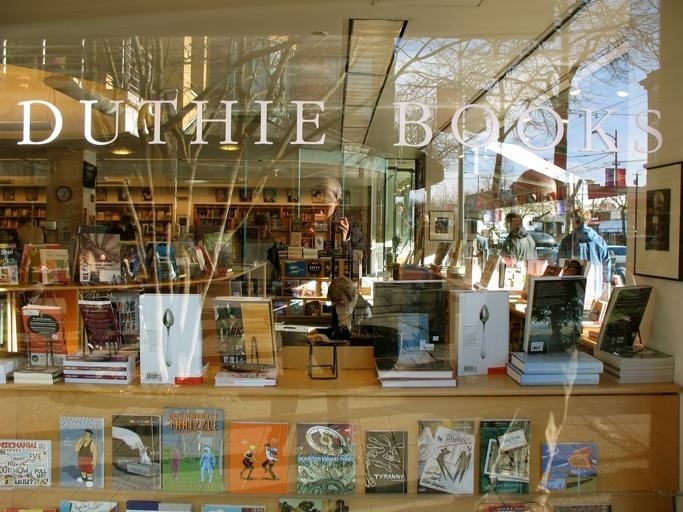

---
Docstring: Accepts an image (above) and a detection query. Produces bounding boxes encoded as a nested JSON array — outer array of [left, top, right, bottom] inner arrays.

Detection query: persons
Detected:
[[254, 214, 280, 282], [267, 174, 365, 296], [310, 276, 372, 342], [487, 228, 504, 248], [477, 170, 613, 508], [12, 216, 46, 254], [557, 209, 611, 284], [643, 191, 669, 251], [476, 220, 488, 248], [111, 213, 136, 241], [502, 213, 538, 261]]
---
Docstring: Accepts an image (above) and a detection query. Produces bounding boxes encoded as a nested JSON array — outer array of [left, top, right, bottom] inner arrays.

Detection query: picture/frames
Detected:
[[634, 161, 683, 280]]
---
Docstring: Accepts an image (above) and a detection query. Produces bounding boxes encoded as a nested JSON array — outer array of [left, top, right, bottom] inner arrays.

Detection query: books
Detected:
[[371, 260, 675, 387], [1, 407, 597, 511], [0, 207, 363, 386], [429, 241, 500, 290]]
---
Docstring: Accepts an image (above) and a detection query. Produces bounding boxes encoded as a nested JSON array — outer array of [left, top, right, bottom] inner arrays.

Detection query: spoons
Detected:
[[163, 308, 174, 367], [479, 305, 489, 359]]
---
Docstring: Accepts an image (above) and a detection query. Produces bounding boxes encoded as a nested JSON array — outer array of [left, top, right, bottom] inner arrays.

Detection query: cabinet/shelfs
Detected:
[[0, 203, 47, 246], [192, 204, 367, 324], [96, 204, 174, 261], [0, 346, 682, 511]]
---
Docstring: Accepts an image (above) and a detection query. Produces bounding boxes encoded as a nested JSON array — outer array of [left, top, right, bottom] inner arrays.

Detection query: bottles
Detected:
[[175, 240, 218, 278]]
